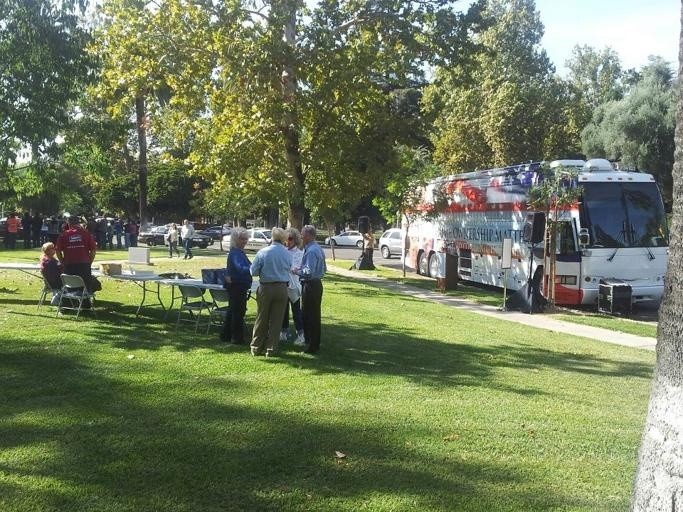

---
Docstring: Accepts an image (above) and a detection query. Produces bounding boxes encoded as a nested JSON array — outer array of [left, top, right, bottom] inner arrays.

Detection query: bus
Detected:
[[401, 156, 669, 309]]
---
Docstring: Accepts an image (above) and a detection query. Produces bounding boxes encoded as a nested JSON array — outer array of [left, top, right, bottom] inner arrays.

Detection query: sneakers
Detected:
[[298, 333, 305, 343], [279, 331, 287, 341]]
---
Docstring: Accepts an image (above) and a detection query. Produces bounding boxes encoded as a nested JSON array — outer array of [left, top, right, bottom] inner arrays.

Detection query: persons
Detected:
[[361, 231, 375, 266], [55, 215, 96, 316], [180, 219, 195, 260], [2, 211, 138, 249], [167, 223, 180, 258], [290, 223, 327, 354], [39, 241, 63, 289], [248, 227, 292, 358], [218, 226, 253, 345], [280, 228, 307, 347]]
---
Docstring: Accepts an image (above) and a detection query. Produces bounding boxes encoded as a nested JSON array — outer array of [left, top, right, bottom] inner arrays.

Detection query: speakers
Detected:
[[358, 215, 369, 233], [523, 212, 545, 242]]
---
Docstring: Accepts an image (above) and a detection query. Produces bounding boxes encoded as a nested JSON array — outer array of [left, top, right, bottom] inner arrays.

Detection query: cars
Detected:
[[323, 230, 375, 249], [95, 215, 273, 250]]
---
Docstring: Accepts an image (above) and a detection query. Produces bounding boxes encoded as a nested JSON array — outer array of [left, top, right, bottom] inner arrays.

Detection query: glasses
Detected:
[[287, 237, 294, 241]]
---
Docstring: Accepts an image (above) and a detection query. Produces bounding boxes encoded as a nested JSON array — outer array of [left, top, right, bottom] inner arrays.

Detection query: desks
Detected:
[[0, 263, 258, 337]]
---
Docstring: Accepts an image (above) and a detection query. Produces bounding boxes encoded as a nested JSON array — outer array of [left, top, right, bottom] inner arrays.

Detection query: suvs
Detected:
[[378, 228, 401, 258]]
